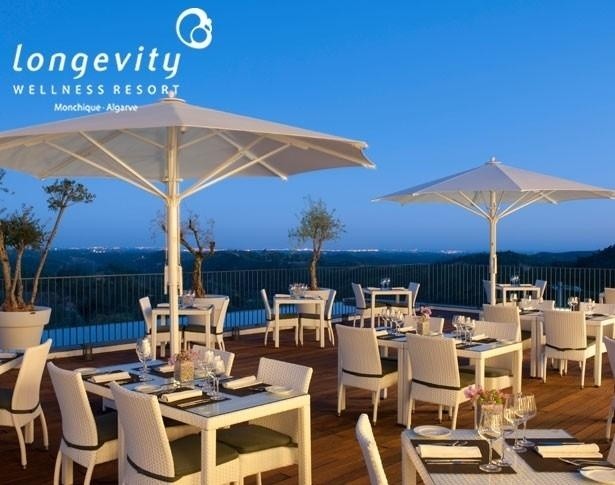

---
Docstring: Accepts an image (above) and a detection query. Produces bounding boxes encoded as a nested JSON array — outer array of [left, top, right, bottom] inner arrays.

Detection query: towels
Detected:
[[534, 442, 603, 459], [416, 445, 483, 459], [0, 353, 17, 359], [157, 303, 169, 307]]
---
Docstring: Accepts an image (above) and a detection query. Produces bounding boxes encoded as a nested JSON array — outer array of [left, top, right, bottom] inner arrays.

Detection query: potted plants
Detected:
[[288, 192, 349, 329], [1, 179, 97, 368], [149, 202, 225, 327]]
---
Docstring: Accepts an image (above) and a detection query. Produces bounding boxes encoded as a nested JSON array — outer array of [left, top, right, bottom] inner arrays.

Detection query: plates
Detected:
[[134, 384, 163, 394], [580, 465, 615, 485], [264, 384, 293, 396], [413, 424, 451, 438], [74, 366, 98, 376]]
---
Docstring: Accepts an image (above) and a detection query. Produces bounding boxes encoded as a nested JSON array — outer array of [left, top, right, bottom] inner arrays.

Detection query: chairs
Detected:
[[48, 345, 314, 485], [607, 433, 615, 467], [183, 296, 230, 351], [355, 413, 388, 485], [139, 297, 182, 353], [0, 338, 52, 469]]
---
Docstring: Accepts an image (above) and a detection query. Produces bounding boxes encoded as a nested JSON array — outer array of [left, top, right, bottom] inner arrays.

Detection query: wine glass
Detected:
[[477, 404, 503, 473], [451, 314, 476, 345], [503, 396, 529, 454], [583, 298, 595, 317], [514, 392, 537, 447], [380, 309, 405, 336], [510, 293, 517, 303], [566, 296, 578, 311], [196, 347, 226, 401], [135, 337, 153, 382], [379, 277, 391, 290], [183, 288, 197, 307], [290, 282, 308, 298], [490, 403, 515, 467]]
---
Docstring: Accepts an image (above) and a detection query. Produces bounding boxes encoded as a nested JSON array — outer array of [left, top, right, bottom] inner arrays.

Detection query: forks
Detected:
[[559, 458, 610, 466], [430, 440, 468, 446]]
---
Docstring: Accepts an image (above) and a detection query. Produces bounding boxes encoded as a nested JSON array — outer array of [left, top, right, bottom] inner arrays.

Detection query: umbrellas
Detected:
[[371, 158, 615, 306], [0, 90, 377, 367]]
